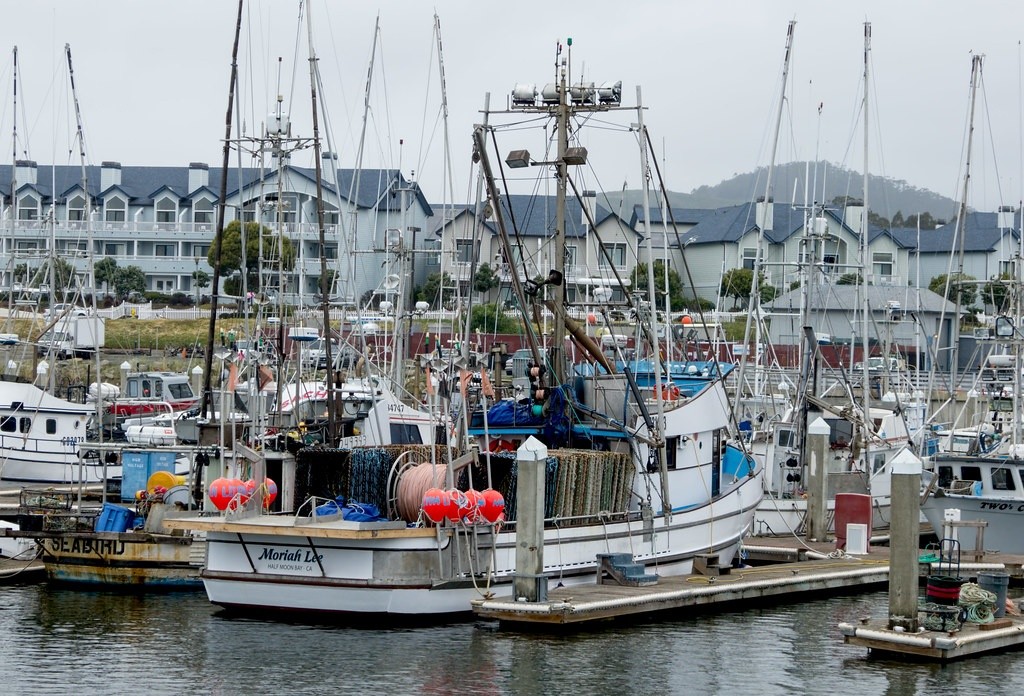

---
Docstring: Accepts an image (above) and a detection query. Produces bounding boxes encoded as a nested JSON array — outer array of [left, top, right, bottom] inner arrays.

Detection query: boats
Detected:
[[3, 0, 1024, 665]]
